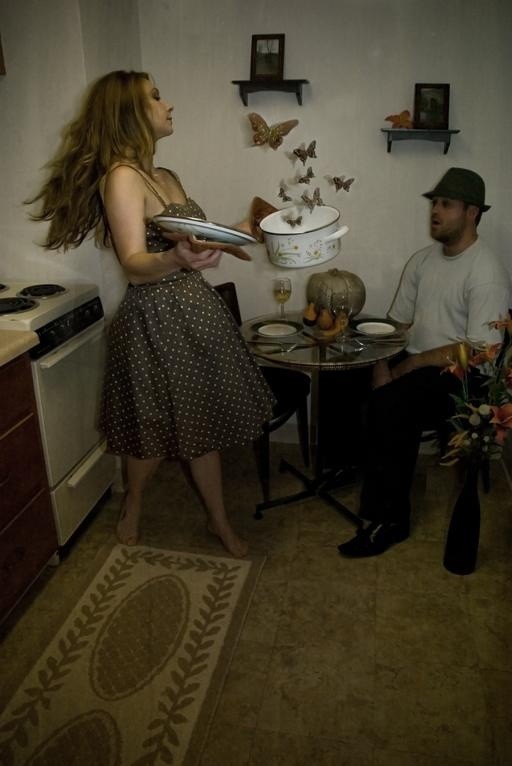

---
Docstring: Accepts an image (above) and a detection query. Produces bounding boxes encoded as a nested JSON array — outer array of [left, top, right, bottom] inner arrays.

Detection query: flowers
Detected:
[[430, 312, 512, 472]]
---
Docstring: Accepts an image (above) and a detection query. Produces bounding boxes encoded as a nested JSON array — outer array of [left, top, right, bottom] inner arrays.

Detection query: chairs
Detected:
[[410, 308, 511, 501], [205, 280, 310, 504]]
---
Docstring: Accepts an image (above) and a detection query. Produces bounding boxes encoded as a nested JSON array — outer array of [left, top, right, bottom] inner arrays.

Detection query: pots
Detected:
[[261, 205, 351, 269]]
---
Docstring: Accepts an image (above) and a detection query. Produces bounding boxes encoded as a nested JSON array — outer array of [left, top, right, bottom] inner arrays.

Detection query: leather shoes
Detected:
[[337, 514, 411, 559]]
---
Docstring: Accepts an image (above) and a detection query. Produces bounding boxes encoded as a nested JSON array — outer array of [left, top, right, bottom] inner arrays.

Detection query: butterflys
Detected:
[[293, 140, 317, 162], [248, 113, 300, 151], [298, 166, 315, 185], [333, 176, 355, 192], [301, 187, 323, 210], [278, 188, 292, 203], [286, 216, 303, 228]]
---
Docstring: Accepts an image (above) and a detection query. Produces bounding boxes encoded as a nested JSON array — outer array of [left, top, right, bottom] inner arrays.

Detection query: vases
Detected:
[[442, 457, 486, 576]]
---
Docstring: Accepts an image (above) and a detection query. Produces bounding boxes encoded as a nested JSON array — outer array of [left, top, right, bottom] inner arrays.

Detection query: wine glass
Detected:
[[273, 277, 292, 319]]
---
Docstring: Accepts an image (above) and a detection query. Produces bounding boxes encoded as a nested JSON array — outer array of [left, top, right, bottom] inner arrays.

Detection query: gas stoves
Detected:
[[0, 277, 107, 359]]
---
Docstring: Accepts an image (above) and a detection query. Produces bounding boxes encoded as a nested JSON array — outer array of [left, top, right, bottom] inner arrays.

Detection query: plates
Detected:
[[349, 317, 402, 338], [251, 319, 304, 339]]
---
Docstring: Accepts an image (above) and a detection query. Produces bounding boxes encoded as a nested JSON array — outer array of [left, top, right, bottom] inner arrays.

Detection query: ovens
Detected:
[[30, 319, 124, 546]]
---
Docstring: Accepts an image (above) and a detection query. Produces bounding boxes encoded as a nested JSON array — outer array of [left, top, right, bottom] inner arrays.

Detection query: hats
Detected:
[[422, 167, 492, 212]]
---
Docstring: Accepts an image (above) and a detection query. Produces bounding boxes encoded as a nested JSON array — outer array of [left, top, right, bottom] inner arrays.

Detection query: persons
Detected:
[[337, 166, 512, 574], [25, 70, 275, 558]]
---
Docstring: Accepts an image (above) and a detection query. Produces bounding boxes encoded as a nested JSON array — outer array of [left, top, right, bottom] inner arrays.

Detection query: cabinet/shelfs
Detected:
[[1, 353, 58, 643]]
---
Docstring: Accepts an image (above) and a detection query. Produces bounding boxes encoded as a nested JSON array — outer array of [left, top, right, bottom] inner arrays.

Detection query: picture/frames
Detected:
[[249, 32, 286, 80], [412, 82, 451, 129]]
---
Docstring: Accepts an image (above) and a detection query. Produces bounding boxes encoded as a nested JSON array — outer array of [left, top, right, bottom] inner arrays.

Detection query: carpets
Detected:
[[1, 536, 270, 766]]
